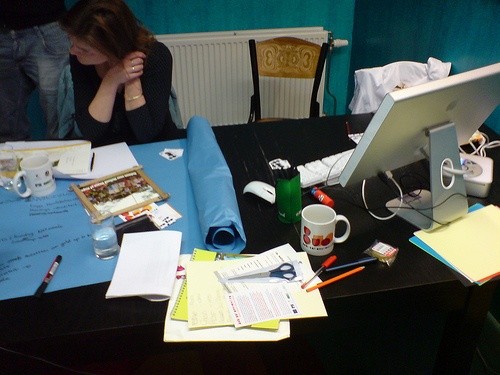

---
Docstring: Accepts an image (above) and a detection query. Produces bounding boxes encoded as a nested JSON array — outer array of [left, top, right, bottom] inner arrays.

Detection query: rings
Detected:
[[132, 66, 135, 70]]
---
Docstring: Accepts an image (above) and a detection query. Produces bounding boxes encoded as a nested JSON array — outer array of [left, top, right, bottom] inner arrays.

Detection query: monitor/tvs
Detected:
[[340, 62, 500, 232]]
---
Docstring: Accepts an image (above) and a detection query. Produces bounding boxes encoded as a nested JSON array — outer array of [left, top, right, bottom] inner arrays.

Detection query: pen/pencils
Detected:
[[306, 266, 365, 293], [345, 120, 350, 141], [89, 151, 95, 171], [300, 255, 336, 288], [34, 254, 62, 298], [324, 257, 379, 272]]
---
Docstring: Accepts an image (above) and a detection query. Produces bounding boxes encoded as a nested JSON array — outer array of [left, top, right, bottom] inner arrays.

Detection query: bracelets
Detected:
[[125, 93, 144, 102]]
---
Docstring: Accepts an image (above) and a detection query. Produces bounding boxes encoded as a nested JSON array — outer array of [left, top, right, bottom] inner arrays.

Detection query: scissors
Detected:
[[228, 263, 296, 281]]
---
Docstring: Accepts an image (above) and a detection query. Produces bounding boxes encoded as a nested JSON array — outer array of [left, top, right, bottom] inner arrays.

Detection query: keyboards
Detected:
[[295, 149, 354, 187]]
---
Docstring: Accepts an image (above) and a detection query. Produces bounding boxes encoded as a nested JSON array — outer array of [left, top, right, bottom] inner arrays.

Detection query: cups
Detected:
[[12, 155, 56, 198], [300, 204, 350, 256], [90, 210, 118, 260], [0, 144, 22, 190]]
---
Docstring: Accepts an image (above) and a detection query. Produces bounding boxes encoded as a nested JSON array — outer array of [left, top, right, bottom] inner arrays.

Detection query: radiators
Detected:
[[150, 27, 349, 130]]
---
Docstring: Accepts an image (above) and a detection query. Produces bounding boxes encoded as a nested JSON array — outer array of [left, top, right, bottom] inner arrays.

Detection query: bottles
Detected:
[[276, 164, 302, 223]]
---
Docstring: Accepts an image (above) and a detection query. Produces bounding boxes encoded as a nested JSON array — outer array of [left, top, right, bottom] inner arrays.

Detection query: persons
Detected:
[[0, 0, 76, 140], [58, 0, 179, 148]]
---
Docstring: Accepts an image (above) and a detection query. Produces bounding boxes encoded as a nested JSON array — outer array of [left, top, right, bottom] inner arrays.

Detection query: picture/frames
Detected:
[[71, 164, 170, 226]]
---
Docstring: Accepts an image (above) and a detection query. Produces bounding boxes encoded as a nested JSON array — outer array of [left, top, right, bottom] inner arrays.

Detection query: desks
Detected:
[[0, 111, 500, 375]]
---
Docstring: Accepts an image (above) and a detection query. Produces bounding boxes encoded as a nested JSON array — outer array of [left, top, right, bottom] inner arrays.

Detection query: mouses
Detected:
[[242, 180, 276, 206]]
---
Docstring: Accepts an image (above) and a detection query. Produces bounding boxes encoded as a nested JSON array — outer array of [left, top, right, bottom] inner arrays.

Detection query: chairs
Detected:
[[246, 37, 330, 125]]
